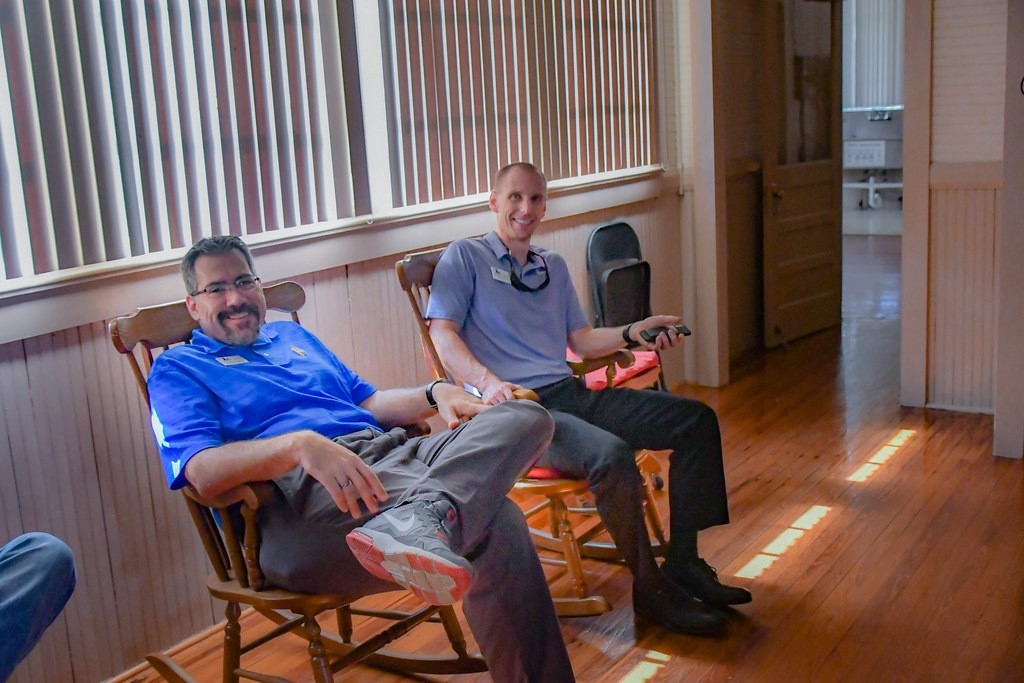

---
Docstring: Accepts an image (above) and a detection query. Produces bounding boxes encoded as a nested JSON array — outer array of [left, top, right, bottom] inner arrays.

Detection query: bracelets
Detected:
[[623, 322, 638, 345]]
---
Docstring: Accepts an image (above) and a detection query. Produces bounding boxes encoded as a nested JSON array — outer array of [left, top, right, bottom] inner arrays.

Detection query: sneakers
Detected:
[[345, 500, 475, 606]]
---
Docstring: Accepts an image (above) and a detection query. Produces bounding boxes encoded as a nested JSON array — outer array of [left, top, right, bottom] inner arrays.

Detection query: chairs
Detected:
[[586, 225, 671, 395], [109, 281, 488, 682], [394, 248, 670, 617], [568, 341, 663, 395]]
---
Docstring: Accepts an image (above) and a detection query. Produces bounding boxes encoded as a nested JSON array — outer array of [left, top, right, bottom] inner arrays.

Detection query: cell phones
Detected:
[[640, 324, 692, 342]]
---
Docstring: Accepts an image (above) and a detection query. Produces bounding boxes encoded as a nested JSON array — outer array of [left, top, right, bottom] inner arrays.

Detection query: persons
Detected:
[[146, 237, 577, 683], [0, 531, 77, 683], [424, 162, 752, 635]]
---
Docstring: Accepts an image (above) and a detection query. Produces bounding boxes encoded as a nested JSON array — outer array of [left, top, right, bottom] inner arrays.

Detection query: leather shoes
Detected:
[[632, 576, 726, 631], [660, 558, 753, 603]]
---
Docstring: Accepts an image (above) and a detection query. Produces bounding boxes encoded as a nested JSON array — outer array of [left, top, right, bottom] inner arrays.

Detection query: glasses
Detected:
[[191, 275, 261, 296], [505, 253, 549, 293]]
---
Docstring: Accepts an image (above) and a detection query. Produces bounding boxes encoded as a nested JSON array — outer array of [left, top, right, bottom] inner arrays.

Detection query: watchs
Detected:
[[426, 377, 448, 410]]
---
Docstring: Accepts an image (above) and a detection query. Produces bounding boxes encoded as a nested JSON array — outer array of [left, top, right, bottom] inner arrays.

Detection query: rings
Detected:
[[341, 481, 352, 488]]
